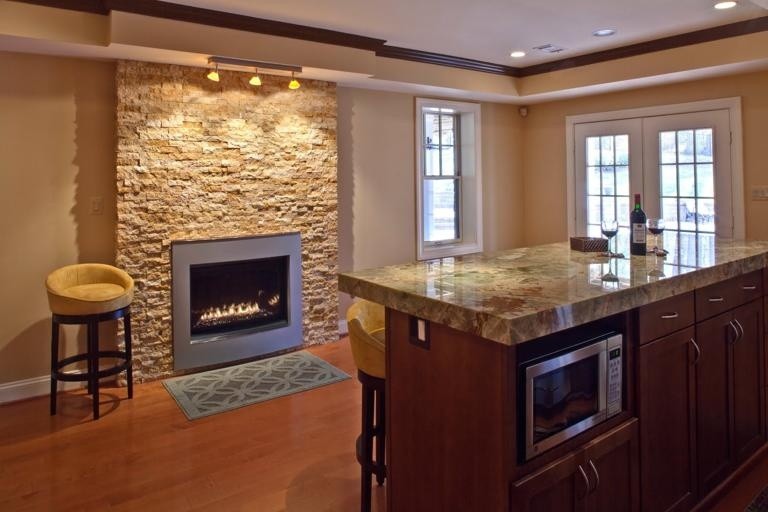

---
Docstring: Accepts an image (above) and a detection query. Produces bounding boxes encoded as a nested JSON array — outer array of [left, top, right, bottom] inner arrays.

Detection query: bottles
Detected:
[[629, 193, 648, 256]]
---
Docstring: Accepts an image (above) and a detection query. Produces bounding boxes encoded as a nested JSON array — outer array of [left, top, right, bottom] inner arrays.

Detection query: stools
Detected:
[[44, 263, 135, 422], [344, 296, 396, 512]]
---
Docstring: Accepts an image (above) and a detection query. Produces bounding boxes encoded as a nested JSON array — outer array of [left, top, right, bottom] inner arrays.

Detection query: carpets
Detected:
[[161, 348, 351, 422]]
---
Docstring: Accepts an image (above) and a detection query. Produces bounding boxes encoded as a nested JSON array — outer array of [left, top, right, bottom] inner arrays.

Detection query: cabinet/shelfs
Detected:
[[635, 289, 699, 512], [511, 417, 641, 512], [696, 270, 767, 502]]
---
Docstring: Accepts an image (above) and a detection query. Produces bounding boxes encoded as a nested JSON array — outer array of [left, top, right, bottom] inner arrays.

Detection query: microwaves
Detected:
[[516, 329, 625, 464]]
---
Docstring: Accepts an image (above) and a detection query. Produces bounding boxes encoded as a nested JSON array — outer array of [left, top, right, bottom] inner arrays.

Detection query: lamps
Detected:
[[206, 56, 303, 91]]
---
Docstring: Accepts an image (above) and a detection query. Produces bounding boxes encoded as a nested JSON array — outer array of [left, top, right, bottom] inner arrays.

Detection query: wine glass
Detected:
[[600, 219, 619, 257], [648, 253, 665, 277], [645, 217, 665, 253], [601, 256, 621, 283]]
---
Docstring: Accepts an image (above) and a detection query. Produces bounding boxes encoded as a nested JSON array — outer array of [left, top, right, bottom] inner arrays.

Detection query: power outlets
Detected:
[[751, 185, 768, 200]]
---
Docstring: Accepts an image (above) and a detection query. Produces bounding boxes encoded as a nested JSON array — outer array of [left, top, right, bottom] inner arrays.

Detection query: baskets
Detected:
[[569, 236, 608, 253]]
